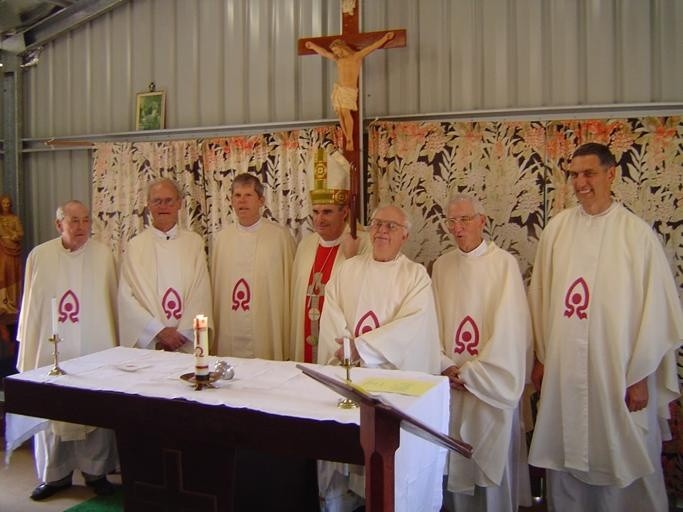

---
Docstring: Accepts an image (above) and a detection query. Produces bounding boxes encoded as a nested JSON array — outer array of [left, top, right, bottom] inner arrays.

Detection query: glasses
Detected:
[[369, 218, 404, 230], [445, 213, 479, 229], [147, 197, 176, 206]]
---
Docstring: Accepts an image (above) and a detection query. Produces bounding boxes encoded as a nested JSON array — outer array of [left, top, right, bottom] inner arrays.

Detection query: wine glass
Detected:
[[180, 371, 221, 390]]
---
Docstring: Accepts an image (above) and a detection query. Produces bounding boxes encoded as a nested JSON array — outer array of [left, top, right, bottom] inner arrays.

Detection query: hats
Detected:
[[308, 143, 351, 206]]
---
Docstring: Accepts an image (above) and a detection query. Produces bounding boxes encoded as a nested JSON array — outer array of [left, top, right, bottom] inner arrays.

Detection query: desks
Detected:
[[1, 346, 451, 511]]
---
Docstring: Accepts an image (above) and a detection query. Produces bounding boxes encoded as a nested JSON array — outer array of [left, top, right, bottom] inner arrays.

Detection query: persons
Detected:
[[315, 200, 445, 511], [303, 31, 396, 151], [288, 192, 375, 365], [208, 173, 298, 359], [0, 195, 27, 316], [528, 142, 682, 509], [429, 192, 535, 511], [12, 199, 122, 501], [115, 174, 218, 356]]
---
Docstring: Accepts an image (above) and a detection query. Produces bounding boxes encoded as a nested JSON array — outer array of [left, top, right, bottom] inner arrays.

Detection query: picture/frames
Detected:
[[134, 90, 167, 132]]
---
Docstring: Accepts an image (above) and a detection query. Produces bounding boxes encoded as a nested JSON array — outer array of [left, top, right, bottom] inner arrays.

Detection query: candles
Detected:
[[343, 336, 350, 361], [191, 312, 209, 376], [49, 295, 59, 337]]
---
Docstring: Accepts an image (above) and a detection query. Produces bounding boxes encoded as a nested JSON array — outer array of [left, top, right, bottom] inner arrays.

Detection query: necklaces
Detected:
[[306, 237, 337, 308]]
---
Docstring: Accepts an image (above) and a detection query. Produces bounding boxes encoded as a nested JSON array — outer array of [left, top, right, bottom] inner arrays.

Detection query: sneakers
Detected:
[[30, 471, 74, 500], [84, 477, 118, 496]]
